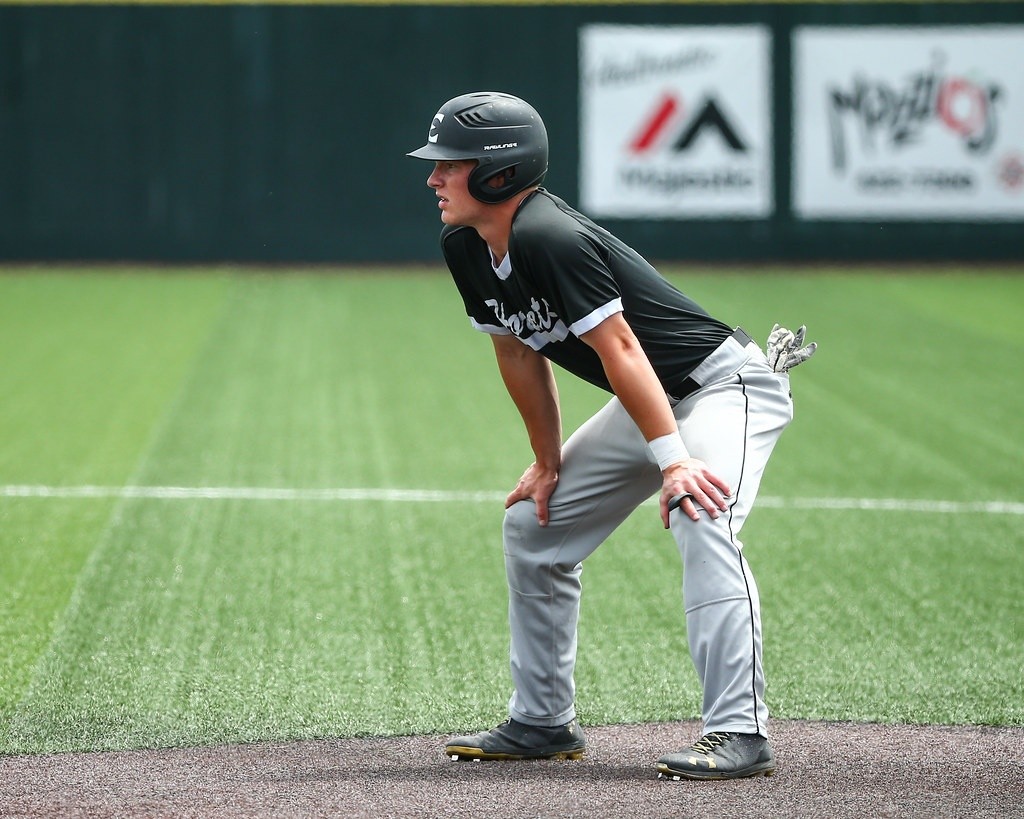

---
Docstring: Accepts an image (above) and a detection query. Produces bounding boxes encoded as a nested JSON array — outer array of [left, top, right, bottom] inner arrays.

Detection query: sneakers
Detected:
[[446, 715, 586, 762], [656, 731, 775, 781]]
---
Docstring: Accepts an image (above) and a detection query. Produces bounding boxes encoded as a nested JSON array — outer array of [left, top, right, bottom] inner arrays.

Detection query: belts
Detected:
[[668, 326, 752, 401]]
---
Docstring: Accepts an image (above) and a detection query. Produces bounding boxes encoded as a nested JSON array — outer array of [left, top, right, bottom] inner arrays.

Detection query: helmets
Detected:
[[406, 92, 549, 204]]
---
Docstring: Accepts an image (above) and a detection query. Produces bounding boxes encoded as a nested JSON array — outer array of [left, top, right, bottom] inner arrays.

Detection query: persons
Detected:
[[407, 92, 818, 780]]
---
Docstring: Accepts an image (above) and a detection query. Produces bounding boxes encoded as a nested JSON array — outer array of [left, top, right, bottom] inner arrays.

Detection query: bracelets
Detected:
[[649, 430, 690, 472]]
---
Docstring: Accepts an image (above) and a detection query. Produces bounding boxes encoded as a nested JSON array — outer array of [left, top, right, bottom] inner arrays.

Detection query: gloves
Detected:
[[768, 322, 817, 373]]
